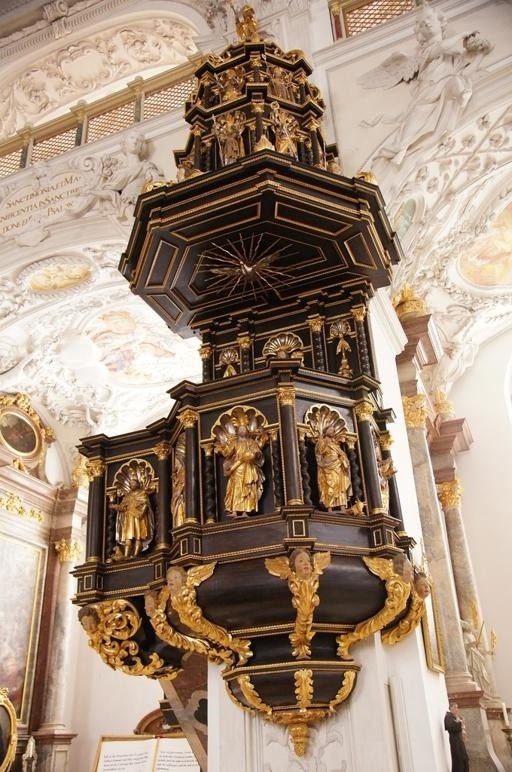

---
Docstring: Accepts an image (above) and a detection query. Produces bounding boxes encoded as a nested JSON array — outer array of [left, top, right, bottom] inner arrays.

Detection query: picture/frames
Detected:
[[0, 526, 49, 729], [0, 687, 18, 772], [0, 407, 41, 459]]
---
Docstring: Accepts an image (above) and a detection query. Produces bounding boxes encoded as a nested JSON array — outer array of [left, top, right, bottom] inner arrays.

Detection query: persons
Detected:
[[86, 130, 165, 222], [143, 590, 158, 617], [288, 549, 314, 579], [109, 479, 154, 559], [392, 552, 414, 584], [166, 566, 187, 591], [413, 572, 429, 598], [372, 7, 494, 167], [314, 425, 353, 513], [444, 701, 470, 771], [78, 607, 99, 633], [214, 426, 266, 518]]
